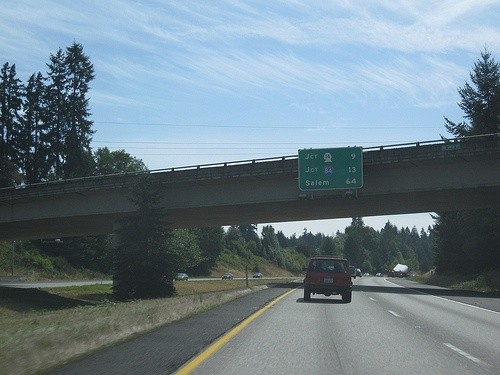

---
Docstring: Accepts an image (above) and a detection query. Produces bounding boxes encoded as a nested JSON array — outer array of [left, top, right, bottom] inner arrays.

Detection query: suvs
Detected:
[[302, 257, 352, 302]]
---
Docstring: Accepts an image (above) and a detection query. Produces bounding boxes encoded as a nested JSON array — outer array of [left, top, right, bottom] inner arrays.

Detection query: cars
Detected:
[[175, 272, 188, 281], [376, 273, 383, 277], [354, 268, 362, 278], [350, 266, 356, 279], [221, 273, 234, 280], [253, 272, 263, 279]]
